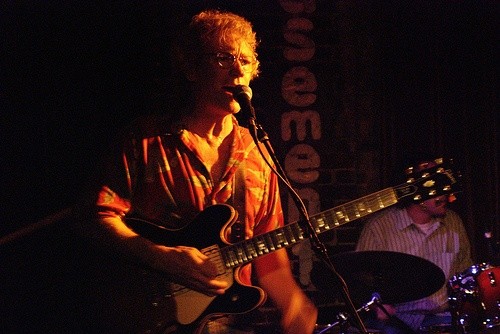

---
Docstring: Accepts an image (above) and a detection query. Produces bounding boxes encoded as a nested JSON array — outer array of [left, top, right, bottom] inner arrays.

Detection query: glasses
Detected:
[[194, 52, 259, 74]]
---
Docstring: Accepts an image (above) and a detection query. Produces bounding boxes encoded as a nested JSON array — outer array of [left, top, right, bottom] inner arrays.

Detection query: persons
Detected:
[[68, 8, 319, 334], [355, 156, 474, 334]]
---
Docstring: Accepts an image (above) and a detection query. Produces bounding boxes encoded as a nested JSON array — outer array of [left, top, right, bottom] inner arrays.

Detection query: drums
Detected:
[[474, 214, 500, 269], [445, 261, 500, 334]]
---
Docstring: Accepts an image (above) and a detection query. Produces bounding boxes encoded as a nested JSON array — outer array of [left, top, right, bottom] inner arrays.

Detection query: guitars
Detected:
[[83, 148, 478, 334]]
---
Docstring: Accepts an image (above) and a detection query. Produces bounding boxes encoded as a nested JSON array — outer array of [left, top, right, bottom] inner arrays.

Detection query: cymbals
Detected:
[[310, 249, 447, 306]]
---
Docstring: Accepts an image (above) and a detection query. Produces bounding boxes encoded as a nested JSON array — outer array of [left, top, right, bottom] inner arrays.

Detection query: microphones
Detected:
[[232, 84, 255, 127]]
